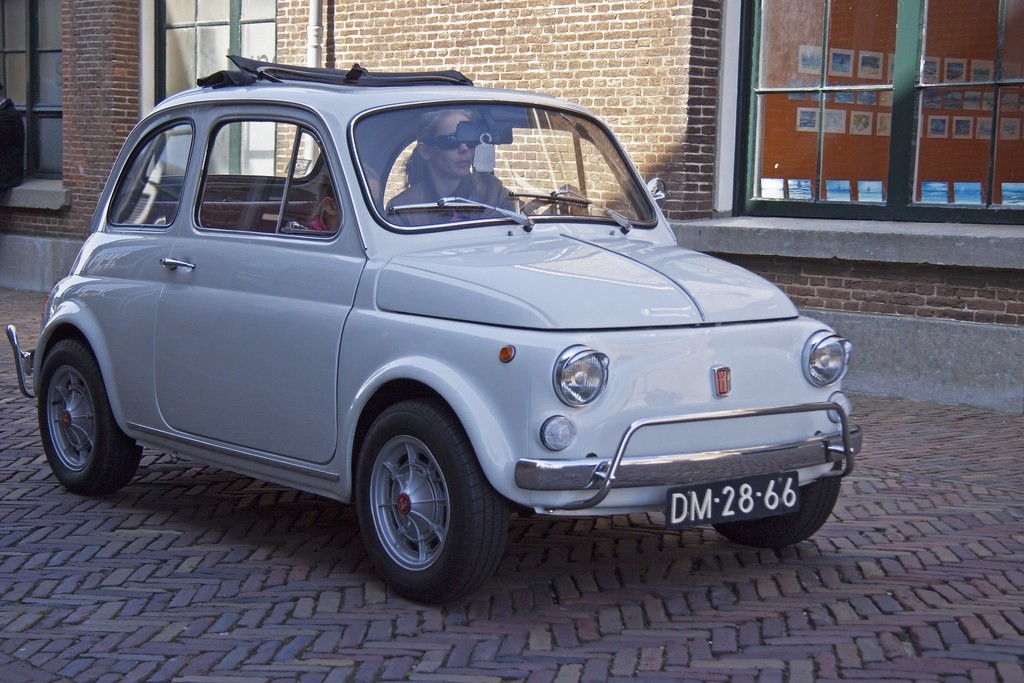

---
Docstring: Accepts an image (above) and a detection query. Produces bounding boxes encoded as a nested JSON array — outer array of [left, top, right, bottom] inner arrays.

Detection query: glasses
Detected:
[[424, 133, 481, 151]]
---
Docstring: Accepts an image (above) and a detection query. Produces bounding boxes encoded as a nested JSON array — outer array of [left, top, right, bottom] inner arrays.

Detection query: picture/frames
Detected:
[[970, 59, 994, 81], [1001, 63, 1021, 88], [798, 45, 823, 74], [824, 109, 846, 134], [834, 83, 854, 104], [999, 118, 1021, 140], [943, 57, 966, 83], [952, 116, 973, 139], [857, 51, 883, 79], [811, 81, 831, 102], [1002, 94, 1019, 112], [963, 91, 982, 110], [887, 53, 894, 82], [927, 116, 949, 138], [849, 111, 873, 135], [916, 89, 942, 109], [788, 80, 806, 100], [917, 56, 940, 83], [857, 91, 876, 106], [876, 113, 891, 137], [828, 48, 854, 77], [944, 91, 961, 109], [976, 117, 992, 139], [878, 91, 893, 106], [796, 107, 819, 132], [982, 92, 994, 111]]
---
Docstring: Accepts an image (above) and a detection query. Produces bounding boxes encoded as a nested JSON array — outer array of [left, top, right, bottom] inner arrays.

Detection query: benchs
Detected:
[[143, 201, 315, 234]]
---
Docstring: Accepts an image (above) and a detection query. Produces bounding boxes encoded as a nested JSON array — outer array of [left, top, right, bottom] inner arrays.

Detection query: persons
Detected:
[[386, 108, 585, 227], [309, 161, 381, 232], [0, 81, 24, 199]]
